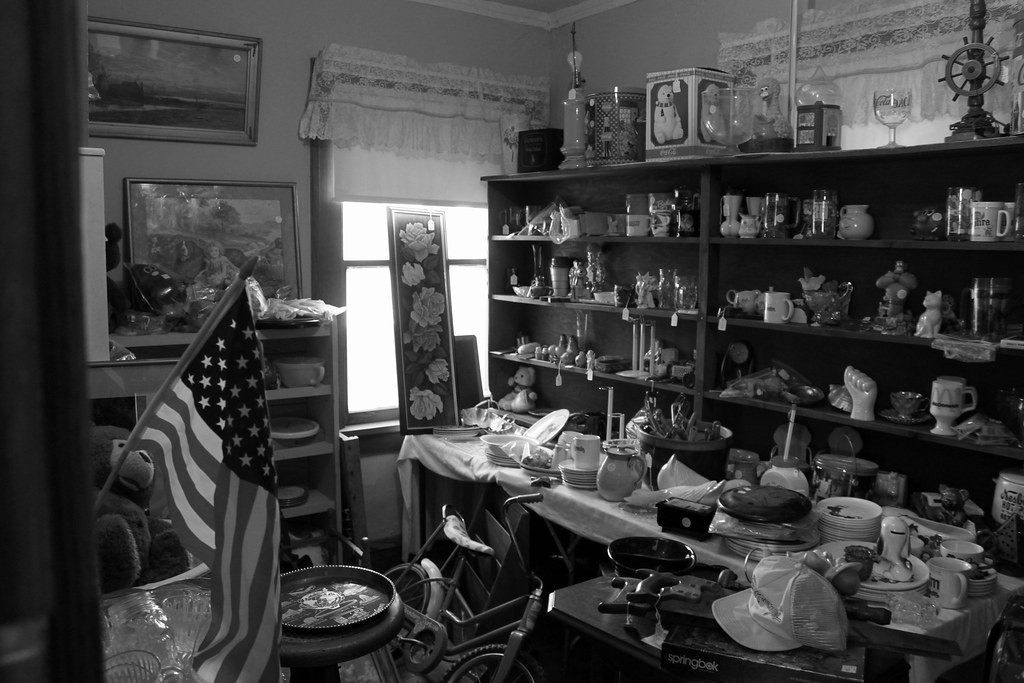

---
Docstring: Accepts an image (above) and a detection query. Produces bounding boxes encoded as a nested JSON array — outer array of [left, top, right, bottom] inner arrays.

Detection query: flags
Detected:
[[124, 275, 282, 683]]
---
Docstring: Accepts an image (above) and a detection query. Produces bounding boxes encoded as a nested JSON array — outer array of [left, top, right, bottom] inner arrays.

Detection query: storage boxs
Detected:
[[517, 128, 564, 171], [645, 68, 734, 162]]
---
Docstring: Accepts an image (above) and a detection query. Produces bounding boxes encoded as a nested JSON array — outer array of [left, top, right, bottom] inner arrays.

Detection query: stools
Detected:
[[261, 591, 404, 683]]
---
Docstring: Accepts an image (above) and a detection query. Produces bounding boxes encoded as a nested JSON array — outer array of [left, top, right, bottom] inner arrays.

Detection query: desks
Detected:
[[402, 409, 1024, 683]]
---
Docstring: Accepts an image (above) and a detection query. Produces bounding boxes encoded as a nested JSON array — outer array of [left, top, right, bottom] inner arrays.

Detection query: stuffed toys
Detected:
[[90, 422, 189, 593], [498, 365, 537, 414]]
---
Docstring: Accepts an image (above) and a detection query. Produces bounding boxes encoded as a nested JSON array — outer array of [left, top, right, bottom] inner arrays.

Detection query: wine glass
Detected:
[[871, 88, 912, 149]]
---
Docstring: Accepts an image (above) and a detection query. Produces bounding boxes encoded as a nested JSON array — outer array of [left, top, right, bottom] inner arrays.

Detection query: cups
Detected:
[[940, 540, 985, 578], [555, 431, 583, 462], [574, 434, 601, 469], [928, 557, 972, 610], [635, 418, 733, 493], [275, 356, 326, 387], [494, 65, 1024, 438], [991, 472, 1024, 526]]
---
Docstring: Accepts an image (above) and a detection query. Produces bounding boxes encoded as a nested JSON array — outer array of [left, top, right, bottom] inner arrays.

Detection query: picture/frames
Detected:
[[85, 14, 262, 147], [386, 205, 464, 436], [453, 335, 484, 413], [124, 174, 306, 302]]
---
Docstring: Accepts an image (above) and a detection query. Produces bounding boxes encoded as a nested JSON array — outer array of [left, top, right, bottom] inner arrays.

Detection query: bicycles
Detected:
[[382, 492, 544, 682]]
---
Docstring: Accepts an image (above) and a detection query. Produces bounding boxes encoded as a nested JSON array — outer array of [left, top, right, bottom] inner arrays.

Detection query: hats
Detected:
[[711, 557, 849, 654]]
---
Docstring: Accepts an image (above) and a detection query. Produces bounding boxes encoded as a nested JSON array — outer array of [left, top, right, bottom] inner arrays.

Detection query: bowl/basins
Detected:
[[269, 417, 320, 450]]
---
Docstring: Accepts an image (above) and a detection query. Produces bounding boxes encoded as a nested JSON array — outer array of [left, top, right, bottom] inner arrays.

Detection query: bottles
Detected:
[[102, 583, 213, 682]]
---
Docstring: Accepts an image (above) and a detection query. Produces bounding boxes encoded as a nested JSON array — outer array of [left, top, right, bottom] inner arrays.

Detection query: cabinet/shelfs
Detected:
[[85, 320, 342, 581], [479, 133, 1024, 576]]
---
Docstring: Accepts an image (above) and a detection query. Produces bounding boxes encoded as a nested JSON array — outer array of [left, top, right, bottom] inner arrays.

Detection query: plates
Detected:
[[433, 407, 999, 652], [278, 486, 309, 508]]
